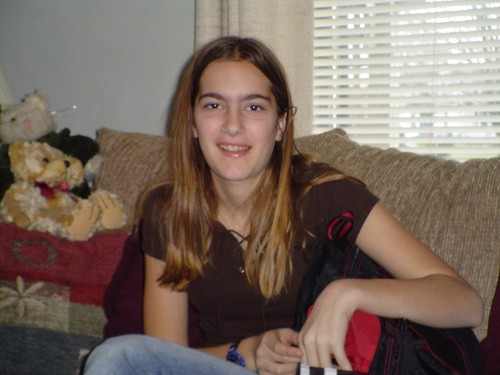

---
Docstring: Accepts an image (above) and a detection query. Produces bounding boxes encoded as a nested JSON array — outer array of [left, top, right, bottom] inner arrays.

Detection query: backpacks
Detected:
[[293, 211, 485, 375]]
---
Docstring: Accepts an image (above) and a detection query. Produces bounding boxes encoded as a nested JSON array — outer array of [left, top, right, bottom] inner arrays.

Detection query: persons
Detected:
[[80, 35, 486, 375]]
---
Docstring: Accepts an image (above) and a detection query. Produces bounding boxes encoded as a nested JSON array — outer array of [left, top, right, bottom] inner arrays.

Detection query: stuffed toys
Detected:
[[0, 86, 129, 243]]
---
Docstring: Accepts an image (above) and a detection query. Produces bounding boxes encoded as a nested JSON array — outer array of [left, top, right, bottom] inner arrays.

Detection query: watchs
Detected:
[[226, 338, 245, 368]]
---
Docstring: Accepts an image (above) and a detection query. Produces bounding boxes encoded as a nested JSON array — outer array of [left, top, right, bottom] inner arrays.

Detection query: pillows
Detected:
[[297, 132, 500, 343], [94, 125, 346, 230], [0, 223, 129, 375]]
